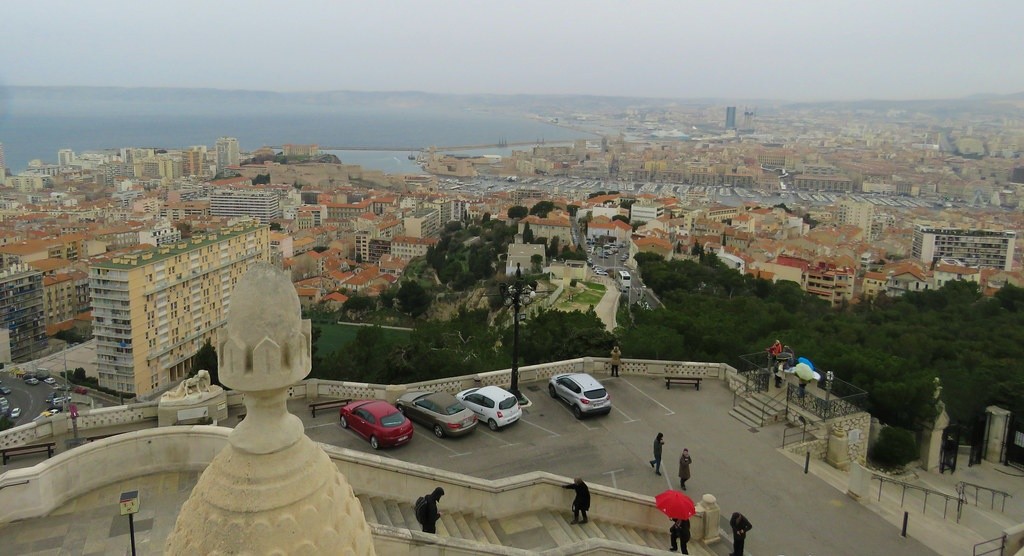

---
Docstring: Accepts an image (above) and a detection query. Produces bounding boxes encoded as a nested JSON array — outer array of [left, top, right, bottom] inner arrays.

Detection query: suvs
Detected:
[[548, 373, 620, 420]]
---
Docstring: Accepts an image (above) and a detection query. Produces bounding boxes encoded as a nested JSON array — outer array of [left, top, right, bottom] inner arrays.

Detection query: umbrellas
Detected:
[[776, 352, 792, 359], [798, 357, 814, 370], [655, 490, 696, 521], [796, 363, 813, 381]]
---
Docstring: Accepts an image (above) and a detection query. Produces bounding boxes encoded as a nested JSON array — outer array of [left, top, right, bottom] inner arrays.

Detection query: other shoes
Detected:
[[650, 461, 654, 467], [670, 548, 676, 551], [656, 472, 661, 476], [578, 520, 588, 524], [571, 520, 578, 524], [681, 484, 686, 490]]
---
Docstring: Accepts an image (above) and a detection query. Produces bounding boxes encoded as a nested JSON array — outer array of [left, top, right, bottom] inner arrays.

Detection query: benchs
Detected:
[[87, 432, 126, 443], [665, 377, 702, 390], [308, 399, 352, 418], [0, 441, 56, 465]]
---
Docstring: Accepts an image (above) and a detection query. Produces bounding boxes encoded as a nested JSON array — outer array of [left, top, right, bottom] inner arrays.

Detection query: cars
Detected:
[[10, 408, 21, 418], [0, 387, 12, 395], [454, 386, 522, 431], [394, 391, 480, 439], [0, 397, 9, 414], [339, 400, 413, 450], [46, 384, 87, 416], [585, 242, 629, 275], [44, 378, 55, 384], [24, 378, 39, 386]]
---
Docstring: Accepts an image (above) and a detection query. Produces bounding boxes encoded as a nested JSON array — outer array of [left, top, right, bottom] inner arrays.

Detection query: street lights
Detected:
[[119, 490, 141, 556], [497, 260, 537, 400], [629, 269, 634, 311]]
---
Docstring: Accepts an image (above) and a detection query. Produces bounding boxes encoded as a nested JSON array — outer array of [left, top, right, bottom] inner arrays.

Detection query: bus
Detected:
[[617, 270, 631, 288]]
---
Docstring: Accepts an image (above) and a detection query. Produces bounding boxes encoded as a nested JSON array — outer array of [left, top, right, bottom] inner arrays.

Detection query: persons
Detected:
[[162, 370, 210, 399], [670, 518, 691, 555], [423, 487, 445, 534], [611, 346, 621, 377], [770, 340, 781, 356], [650, 432, 664, 476], [730, 512, 752, 556], [678, 448, 692, 491], [797, 378, 807, 398], [773, 347, 793, 388], [561, 476, 590, 525]]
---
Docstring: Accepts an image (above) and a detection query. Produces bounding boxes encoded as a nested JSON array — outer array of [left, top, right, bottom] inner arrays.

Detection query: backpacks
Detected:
[[415, 497, 429, 524]]
